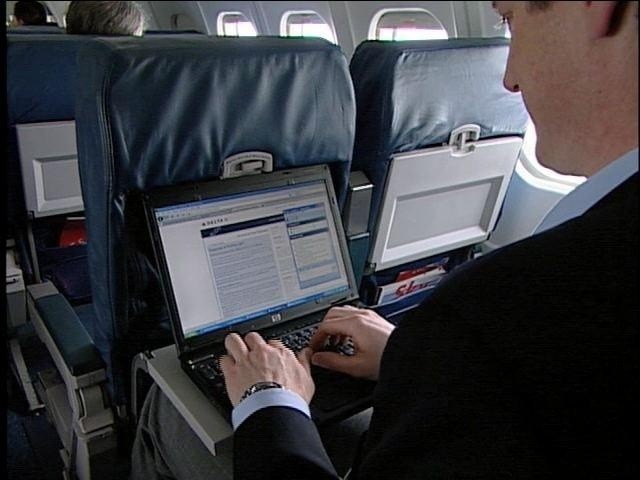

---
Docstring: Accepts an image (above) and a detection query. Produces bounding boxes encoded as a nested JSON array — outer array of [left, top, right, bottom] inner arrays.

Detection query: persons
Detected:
[[9, 0, 48, 27], [65, 1, 146, 38], [126, 1, 640, 479]]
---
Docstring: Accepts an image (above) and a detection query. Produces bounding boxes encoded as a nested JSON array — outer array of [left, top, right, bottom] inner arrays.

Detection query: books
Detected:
[[397, 257, 450, 282], [57, 216, 88, 249], [375, 265, 447, 305]]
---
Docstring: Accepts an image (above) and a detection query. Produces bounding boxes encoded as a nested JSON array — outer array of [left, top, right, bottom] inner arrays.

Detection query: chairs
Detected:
[[7, 26, 529, 480]]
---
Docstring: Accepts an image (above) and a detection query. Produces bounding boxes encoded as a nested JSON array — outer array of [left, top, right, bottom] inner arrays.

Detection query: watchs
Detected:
[[236, 382, 282, 403]]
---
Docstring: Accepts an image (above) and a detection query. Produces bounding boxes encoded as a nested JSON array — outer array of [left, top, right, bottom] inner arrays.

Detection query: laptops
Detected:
[[142, 163, 380, 428]]
